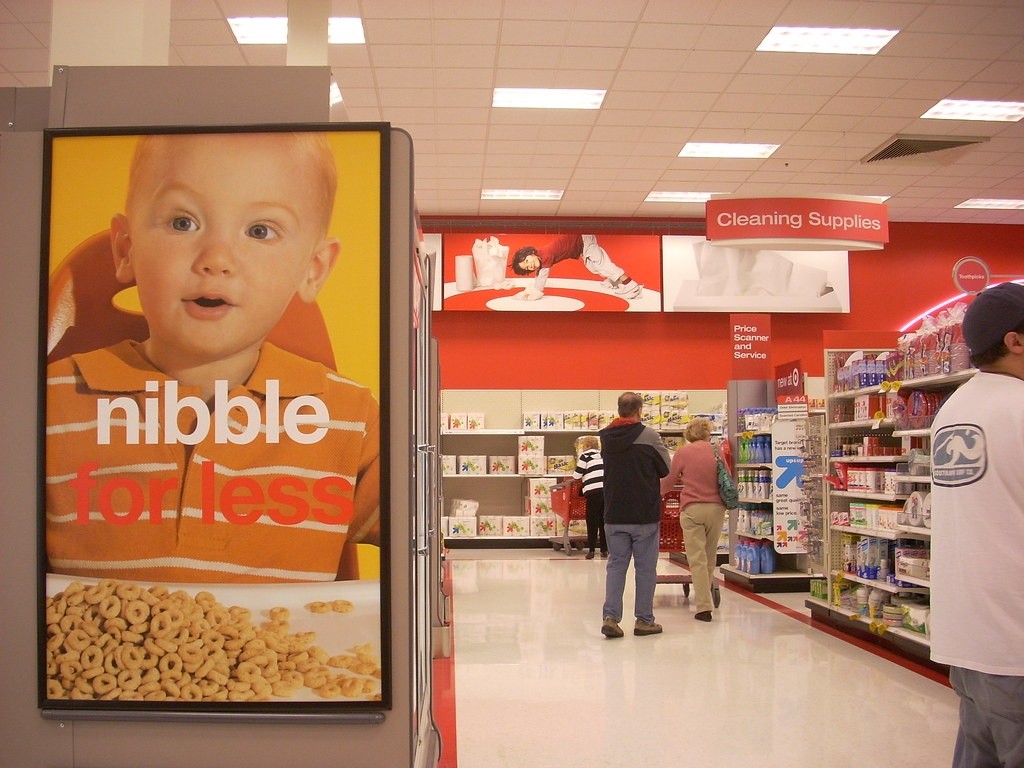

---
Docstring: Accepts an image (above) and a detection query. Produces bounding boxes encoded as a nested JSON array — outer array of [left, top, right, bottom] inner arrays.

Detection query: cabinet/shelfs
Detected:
[[802, 348, 980, 677], [440, 428, 722, 539], [720, 408, 827, 579]]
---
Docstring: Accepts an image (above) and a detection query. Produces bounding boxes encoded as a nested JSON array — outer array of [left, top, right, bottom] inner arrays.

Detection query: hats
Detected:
[[963, 282, 1024, 354]]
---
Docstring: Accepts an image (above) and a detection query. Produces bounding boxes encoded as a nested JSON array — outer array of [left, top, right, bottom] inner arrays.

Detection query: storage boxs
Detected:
[[854, 390, 930, 456], [841, 533, 929, 588]]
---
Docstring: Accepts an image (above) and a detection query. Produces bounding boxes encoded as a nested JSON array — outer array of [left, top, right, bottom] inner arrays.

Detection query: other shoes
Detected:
[[585, 551, 594, 559], [614, 280, 643, 300], [600, 552, 608, 560], [601, 279, 619, 289], [695, 611, 712, 622]]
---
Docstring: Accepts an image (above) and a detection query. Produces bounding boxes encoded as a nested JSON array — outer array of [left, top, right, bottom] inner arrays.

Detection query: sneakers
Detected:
[[601, 618, 624, 637], [634, 620, 662, 635]]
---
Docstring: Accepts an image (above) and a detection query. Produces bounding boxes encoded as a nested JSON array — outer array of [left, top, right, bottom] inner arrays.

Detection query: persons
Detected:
[[46, 130, 381, 587], [660, 418, 726, 622], [573, 436, 609, 560], [930, 281, 1024, 768], [512, 234, 642, 302], [599, 393, 671, 637]]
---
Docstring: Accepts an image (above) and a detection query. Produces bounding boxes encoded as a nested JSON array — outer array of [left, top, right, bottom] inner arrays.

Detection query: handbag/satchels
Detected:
[[712, 443, 739, 510], [579, 484, 585, 496]]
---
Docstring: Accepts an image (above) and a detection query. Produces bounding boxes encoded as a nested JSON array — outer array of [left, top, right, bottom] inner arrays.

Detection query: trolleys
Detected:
[[655, 490, 721, 608], [546, 478, 589, 556]]
